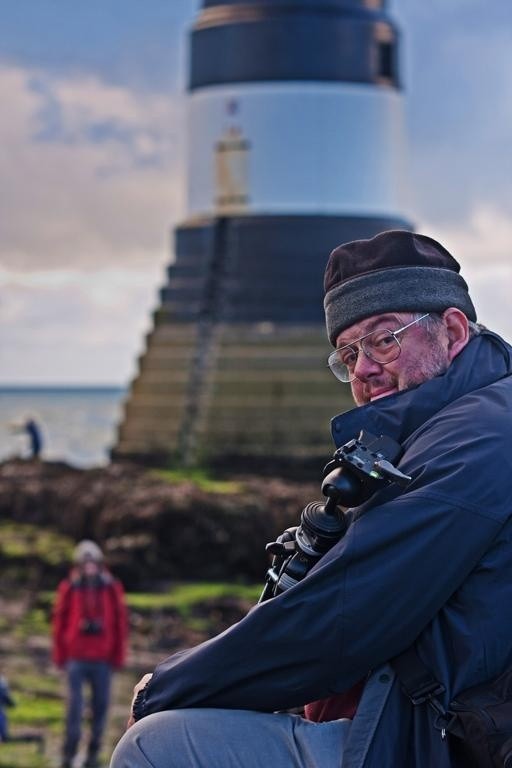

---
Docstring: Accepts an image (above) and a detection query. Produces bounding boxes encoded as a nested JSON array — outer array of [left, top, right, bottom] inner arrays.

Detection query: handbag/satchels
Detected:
[[445, 666, 512, 768]]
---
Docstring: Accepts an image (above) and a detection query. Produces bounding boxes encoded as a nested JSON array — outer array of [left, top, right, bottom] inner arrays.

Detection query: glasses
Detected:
[[327, 312, 430, 383]]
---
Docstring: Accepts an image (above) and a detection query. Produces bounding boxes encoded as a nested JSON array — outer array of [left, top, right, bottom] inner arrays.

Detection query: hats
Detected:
[[73, 540, 103, 564], [323, 230, 477, 348]]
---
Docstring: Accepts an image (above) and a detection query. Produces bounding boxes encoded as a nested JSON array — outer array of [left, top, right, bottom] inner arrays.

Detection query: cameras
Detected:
[[80, 620, 101, 635], [260, 429, 403, 603]]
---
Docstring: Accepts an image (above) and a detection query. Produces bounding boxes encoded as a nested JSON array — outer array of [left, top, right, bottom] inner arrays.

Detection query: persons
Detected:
[[51, 540, 129, 768], [24, 420, 43, 461], [111, 231, 512, 768]]
